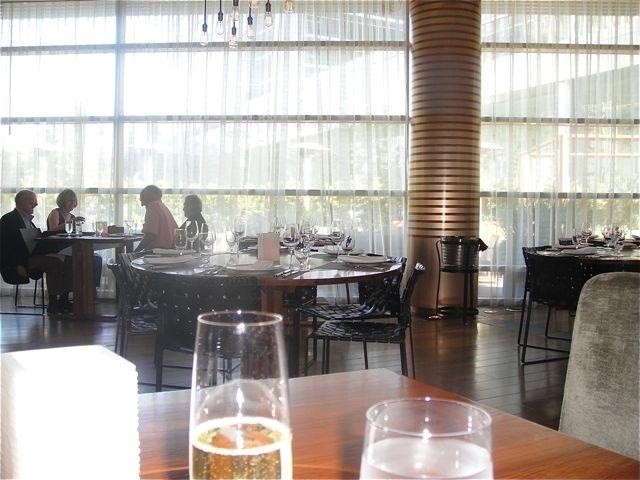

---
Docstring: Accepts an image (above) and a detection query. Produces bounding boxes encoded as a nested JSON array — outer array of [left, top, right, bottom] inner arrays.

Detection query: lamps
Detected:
[[200, 0, 296, 49]]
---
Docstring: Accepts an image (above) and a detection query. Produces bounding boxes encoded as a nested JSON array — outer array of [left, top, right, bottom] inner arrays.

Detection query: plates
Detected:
[[153, 247, 195, 254], [341, 256, 390, 268], [564, 249, 597, 257], [555, 245, 586, 249], [145, 254, 192, 265], [321, 246, 365, 255]]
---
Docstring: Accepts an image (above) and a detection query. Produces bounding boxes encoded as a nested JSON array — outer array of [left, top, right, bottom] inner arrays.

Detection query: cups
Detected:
[[359, 398, 493, 480], [188, 310, 295, 480]]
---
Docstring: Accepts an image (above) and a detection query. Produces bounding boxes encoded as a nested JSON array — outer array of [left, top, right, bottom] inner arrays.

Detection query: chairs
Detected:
[[557, 272, 640, 461], [517, 244, 631, 365]]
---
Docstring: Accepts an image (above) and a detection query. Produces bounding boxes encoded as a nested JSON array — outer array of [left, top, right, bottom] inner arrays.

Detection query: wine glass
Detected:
[[65, 219, 137, 239], [172, 220, 354, 275], [601, 224, 625, 259]]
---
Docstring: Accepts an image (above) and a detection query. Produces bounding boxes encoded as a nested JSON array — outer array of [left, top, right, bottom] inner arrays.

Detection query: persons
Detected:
[[128, 185, 179, 315], [0, 190, 75, 316], [179, 195, 209, 251], [47, 189, 102, 287]]
